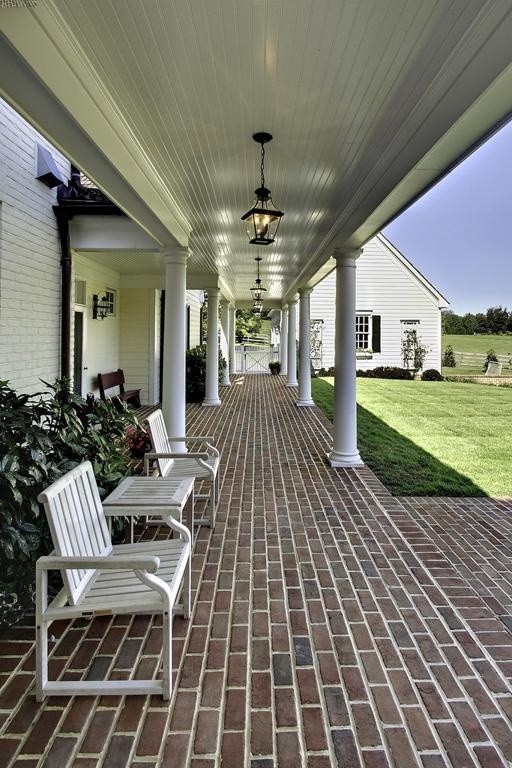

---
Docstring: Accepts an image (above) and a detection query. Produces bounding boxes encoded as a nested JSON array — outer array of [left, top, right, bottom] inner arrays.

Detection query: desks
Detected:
[[101, 475, 196, 539]]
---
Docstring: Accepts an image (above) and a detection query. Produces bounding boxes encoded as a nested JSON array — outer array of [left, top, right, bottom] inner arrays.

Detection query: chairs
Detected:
[[34, 460, 192, 703], [143, 408, 221, 530]]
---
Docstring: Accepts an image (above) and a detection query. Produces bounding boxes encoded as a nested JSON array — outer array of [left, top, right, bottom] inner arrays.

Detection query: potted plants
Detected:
[[269, 362, 279, 375], [185, 344, 227, 403]]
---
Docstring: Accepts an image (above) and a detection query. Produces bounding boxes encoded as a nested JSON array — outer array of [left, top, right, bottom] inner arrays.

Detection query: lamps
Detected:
[[92, 294, 110, 320], [249, 258, 268, 319], [240, 131, 285, 246]]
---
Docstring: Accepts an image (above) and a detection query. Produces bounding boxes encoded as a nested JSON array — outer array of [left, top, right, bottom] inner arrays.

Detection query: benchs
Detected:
[[97, 368, 141, 415]]
